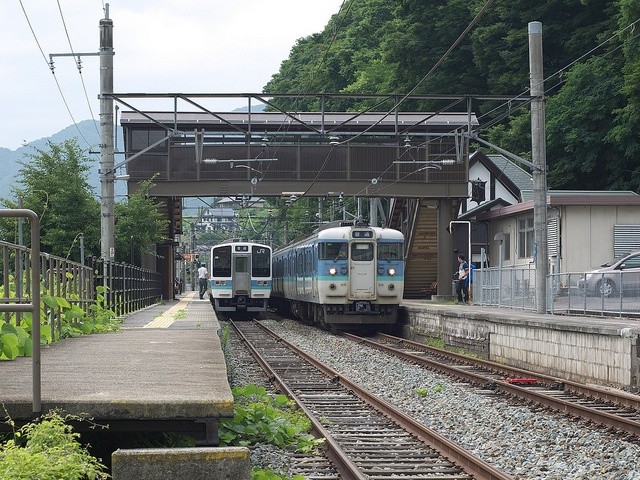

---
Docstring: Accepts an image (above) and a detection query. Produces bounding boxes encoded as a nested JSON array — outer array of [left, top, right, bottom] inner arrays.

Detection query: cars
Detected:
[[576, 249, 640, 298]]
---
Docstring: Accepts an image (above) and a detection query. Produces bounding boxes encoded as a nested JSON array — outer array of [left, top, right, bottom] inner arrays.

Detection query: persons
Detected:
[[466, 264, 475, 302], [454, 254, 470, 306], [198, 263, 209, 300]]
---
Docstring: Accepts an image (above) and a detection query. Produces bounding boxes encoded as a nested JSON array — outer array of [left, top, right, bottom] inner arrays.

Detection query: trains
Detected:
[[208, 237, 272, 321], [272, 215, 408, 334]]
